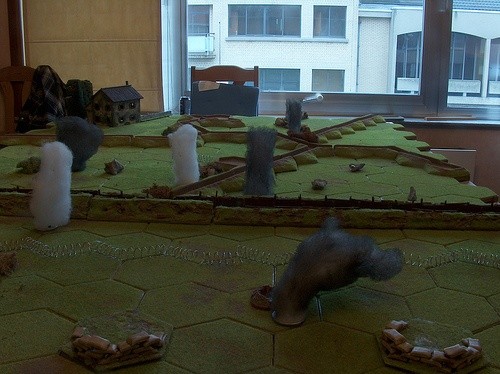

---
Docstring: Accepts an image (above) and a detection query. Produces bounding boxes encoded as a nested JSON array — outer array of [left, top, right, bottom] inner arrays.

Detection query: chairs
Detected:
[[191, 66, 259, 116], [0, 66, 35, 131]]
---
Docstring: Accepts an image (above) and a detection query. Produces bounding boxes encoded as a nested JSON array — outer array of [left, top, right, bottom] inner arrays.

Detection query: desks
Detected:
[[0, 115, 500, 374]]
